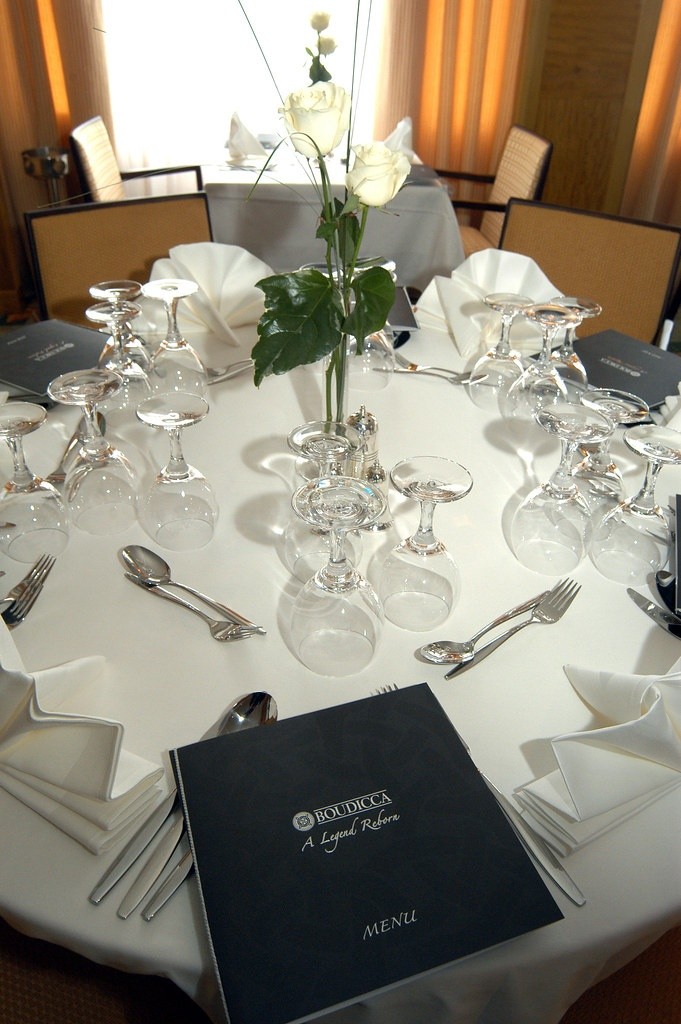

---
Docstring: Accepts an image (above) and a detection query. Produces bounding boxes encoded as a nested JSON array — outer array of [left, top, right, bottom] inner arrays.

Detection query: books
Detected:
[[0, 316, 115, 397], [166, 682, 566, 1024], [528, 328, 681, 412]]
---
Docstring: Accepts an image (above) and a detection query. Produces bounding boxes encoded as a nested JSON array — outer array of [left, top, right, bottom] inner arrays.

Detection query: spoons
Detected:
[[122, 545, 266, 636], [656, 570, 681, 620], [89, 692, 278, 904], [375, 368, 472, 383], [420, 591, 552, 663]]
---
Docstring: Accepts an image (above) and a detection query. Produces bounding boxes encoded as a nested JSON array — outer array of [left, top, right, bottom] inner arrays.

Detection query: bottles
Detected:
[[346, 404, 379, 478]]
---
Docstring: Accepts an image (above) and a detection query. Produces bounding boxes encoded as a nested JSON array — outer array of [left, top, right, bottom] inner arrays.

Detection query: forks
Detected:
[[395, 351, 459, 375], [445, 578, 581, 680], [124, 571, 262, 641], [0, 580, 44, 626], [207, 358, 251, 375], [0, 554, 56, 603]]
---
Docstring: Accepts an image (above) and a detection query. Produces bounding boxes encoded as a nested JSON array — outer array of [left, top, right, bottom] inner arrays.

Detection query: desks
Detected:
[[205, 148, 447, 265], [0, 291, 681, 1024]]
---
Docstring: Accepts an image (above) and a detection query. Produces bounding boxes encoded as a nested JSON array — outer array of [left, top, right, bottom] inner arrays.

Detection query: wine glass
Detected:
[[282, 421, 363, 583], [299, 257, 397, 391], [291, 476, 384, 677], [468, 292, 681, 580], [0, 278, 218, 563], [379, 456, 473, 631]]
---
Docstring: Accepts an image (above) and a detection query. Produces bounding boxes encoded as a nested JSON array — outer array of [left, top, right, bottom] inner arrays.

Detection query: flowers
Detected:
[[238, 0, 415, 420]]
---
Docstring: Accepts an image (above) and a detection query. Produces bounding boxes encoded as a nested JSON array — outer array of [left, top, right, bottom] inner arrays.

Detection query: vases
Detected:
[[291, 261, 396, 481]]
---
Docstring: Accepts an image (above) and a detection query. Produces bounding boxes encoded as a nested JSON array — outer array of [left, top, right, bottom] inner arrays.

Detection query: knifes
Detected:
[[627, 588, 681, 640]]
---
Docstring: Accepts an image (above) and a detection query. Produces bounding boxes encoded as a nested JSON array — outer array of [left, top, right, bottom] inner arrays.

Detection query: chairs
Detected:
[[58, 114, 207, 201], [23, 193, 222, 323], [496, 196, 681, 347], [434, 123, 553, 258]]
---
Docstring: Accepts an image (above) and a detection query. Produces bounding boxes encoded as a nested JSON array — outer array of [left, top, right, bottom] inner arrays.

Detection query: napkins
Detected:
[[415, 250, 578, 365], [0, 615, 165, 855], [513, 656, 681, 857], [650, 381, 681, 429], [130, 243, 279, 348]]
